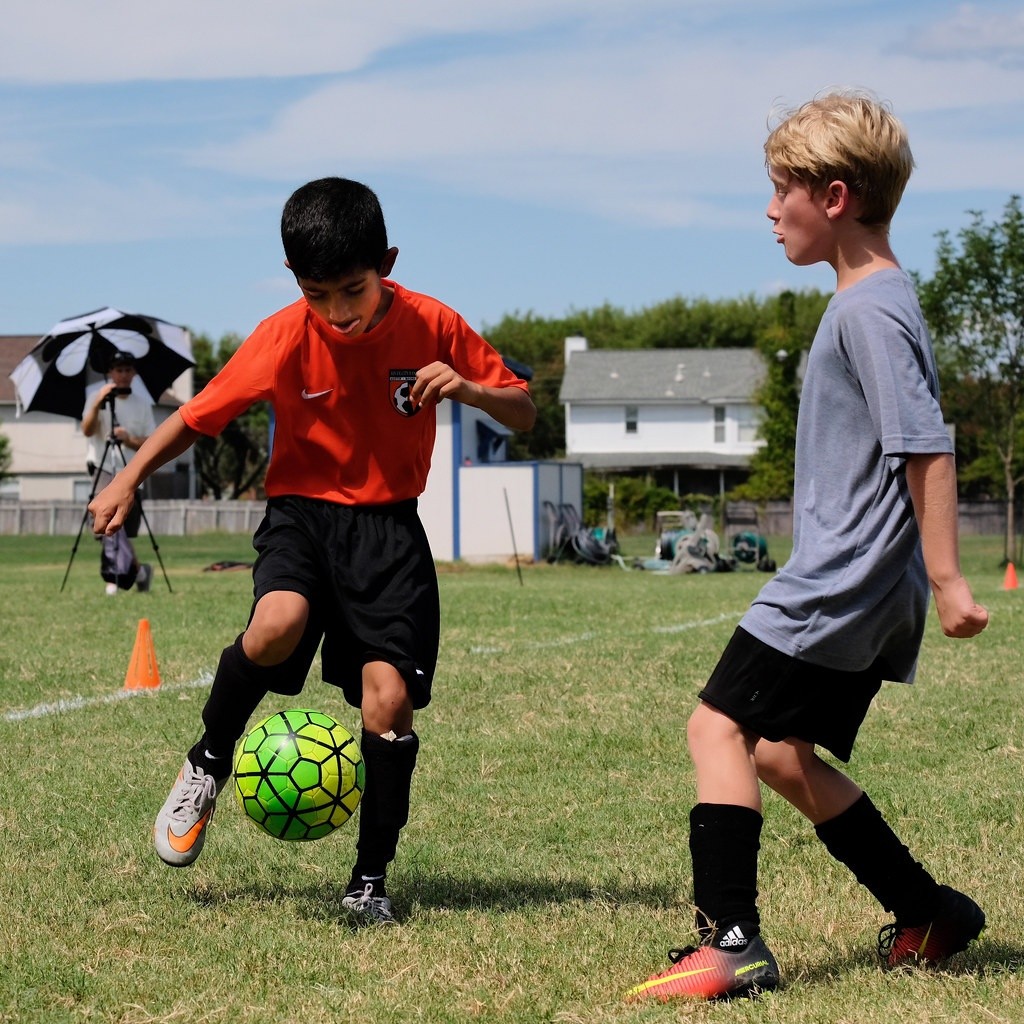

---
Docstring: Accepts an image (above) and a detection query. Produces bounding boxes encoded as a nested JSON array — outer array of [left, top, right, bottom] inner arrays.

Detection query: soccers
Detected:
[[232, 707, 367, 845]]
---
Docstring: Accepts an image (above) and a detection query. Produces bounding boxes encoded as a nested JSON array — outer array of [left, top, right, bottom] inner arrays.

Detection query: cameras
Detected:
[[108, 387, 132, 398]]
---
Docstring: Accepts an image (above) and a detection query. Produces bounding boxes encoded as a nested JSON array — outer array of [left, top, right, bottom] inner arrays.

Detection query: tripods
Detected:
[[60, 398, 173, 593]]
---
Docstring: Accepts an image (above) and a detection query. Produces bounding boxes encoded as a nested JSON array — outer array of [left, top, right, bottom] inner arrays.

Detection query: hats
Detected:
[[111, 350, 137, 365]]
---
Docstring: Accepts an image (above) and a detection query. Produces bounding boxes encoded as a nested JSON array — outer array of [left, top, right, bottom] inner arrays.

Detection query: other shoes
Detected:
[[105, 584, 119, 595], [136, 563, 154, 592]]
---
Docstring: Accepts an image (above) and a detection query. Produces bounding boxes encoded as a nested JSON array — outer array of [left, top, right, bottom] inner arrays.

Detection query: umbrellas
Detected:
[[7, 307, 197, 429]]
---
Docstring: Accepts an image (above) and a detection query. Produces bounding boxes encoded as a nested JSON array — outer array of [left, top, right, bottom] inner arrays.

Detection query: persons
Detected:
[[81, 351, 157, 596], [623, 92, 990, 1000], [86, 176, 540, 921]]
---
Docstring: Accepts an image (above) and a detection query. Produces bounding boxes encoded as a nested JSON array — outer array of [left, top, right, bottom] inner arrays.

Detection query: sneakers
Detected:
[[152, 756, 229, 867], [622, 934, 779, 1005], [340, 882, 395, 921], [877, 884, 985, 973]]
